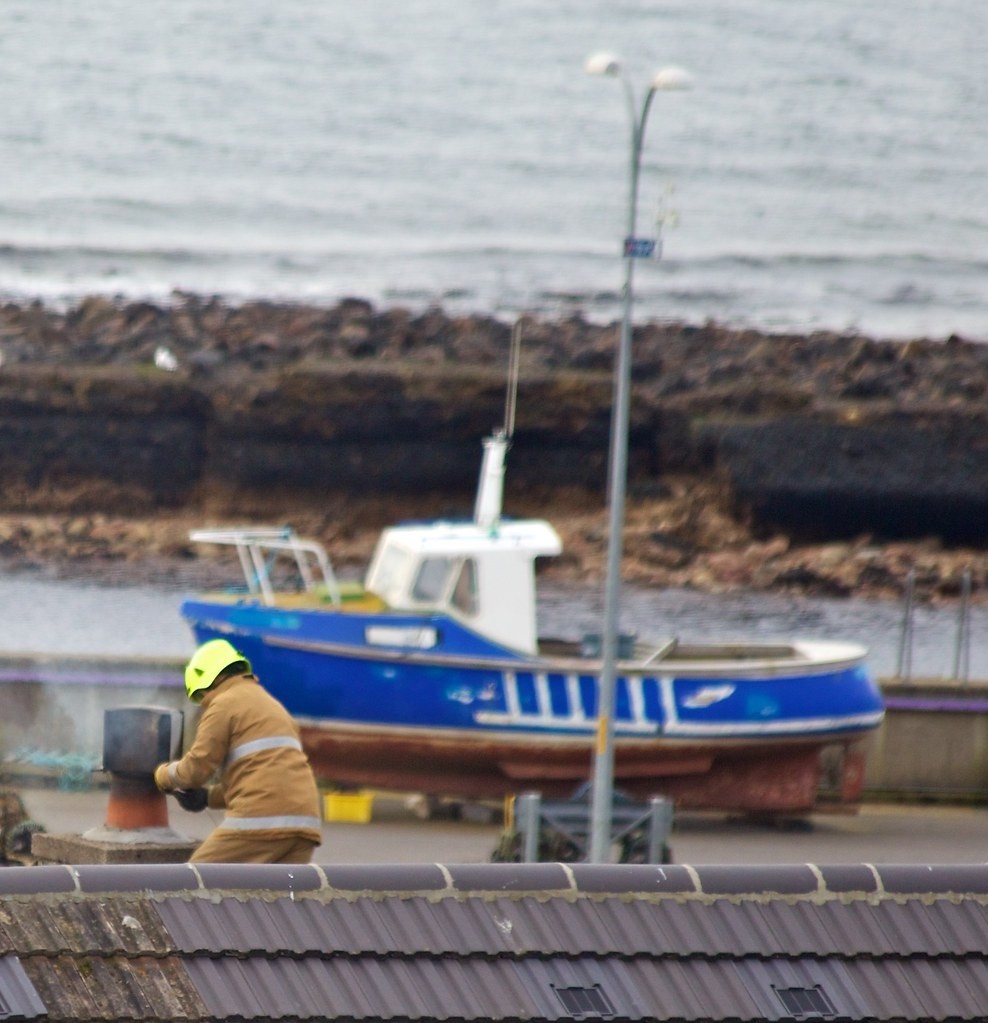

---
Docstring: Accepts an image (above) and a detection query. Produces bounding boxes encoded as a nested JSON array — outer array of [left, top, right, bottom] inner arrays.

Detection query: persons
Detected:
[[153, 640, 324, 864]]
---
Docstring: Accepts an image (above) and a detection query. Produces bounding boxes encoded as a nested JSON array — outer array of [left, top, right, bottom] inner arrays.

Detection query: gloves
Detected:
[[172, 788, 210, 812], [154, 764, 174, 796]]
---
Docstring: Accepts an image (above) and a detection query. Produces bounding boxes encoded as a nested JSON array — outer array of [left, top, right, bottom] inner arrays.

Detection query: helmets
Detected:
[[184, 639, 251, 704]]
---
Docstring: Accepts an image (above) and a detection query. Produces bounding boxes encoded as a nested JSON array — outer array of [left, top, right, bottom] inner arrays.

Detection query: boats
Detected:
[[177, 322, 885, 829]]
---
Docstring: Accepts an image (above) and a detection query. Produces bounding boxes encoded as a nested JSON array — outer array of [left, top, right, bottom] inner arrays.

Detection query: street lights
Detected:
[[585, 49, 698, 865]]
